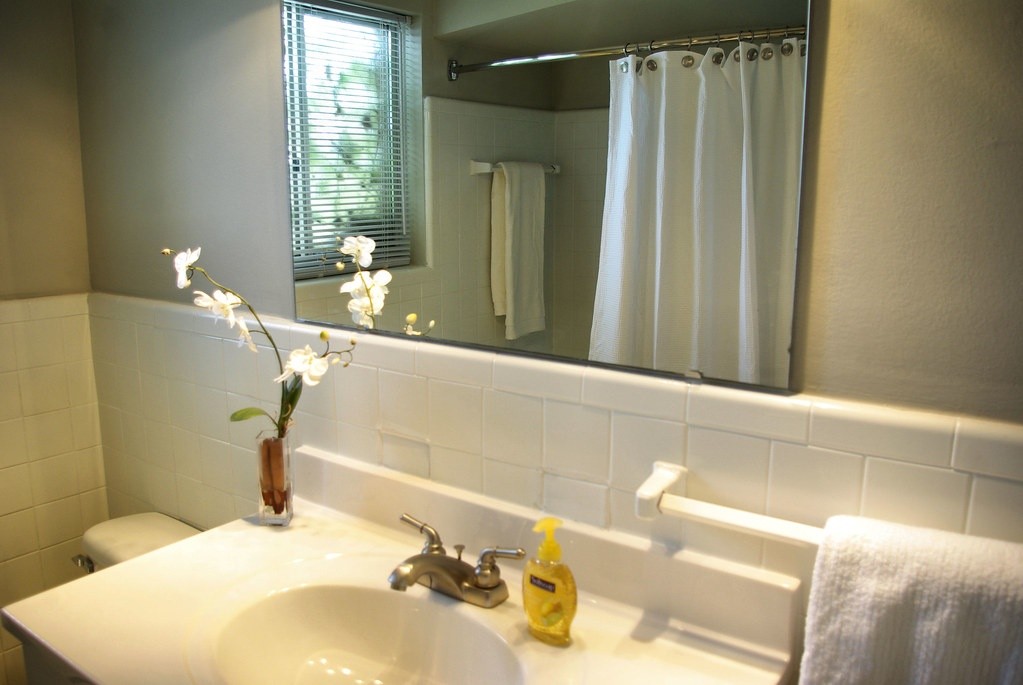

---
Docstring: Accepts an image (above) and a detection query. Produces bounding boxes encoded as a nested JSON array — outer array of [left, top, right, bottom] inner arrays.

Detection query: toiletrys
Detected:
[[520, 516, 578, 649]]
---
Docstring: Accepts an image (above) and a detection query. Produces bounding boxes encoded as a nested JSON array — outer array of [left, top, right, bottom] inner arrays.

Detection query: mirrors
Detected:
[[279, 0, 811, 397]]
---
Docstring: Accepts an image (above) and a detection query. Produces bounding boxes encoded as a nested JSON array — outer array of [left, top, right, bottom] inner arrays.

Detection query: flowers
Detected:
[[160, 245, 357, 436], [322, 234, 436, 335]]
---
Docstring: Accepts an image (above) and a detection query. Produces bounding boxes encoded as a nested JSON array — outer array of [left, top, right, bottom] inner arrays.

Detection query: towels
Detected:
[[491, 161, 546, 340], [796, 514, 1023, 685]]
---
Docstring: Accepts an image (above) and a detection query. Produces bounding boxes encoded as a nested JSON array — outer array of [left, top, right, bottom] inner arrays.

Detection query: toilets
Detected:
[[70, 511, 203, 575]]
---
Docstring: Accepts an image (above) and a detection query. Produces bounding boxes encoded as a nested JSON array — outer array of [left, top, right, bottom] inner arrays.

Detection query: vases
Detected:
[[256, 426, 294, 527]]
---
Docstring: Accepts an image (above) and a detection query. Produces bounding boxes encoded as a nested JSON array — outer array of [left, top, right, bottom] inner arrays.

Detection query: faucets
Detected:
[[386, 512, 528, 609]]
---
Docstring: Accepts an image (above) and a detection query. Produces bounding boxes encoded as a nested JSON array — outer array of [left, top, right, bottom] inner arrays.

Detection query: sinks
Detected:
[[214, 583, 525, 685]]
